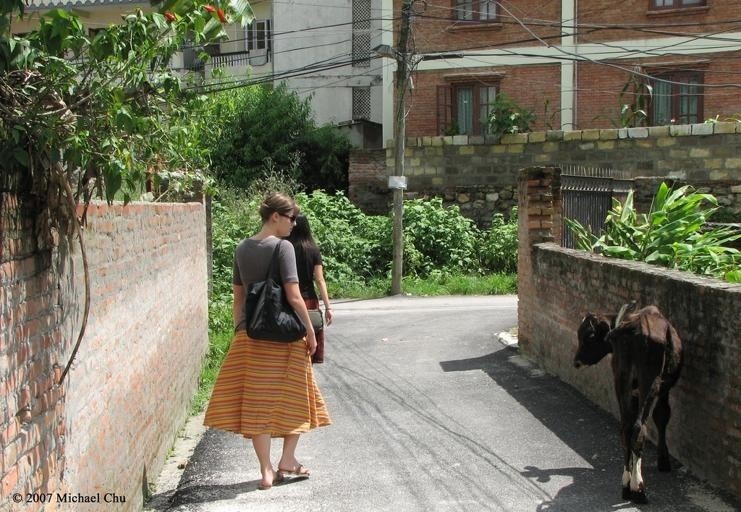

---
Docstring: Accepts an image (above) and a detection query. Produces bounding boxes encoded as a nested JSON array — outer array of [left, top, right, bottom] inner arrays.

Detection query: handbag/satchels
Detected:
[[295, 307, 324, 334], [246, 277, 308, 343]]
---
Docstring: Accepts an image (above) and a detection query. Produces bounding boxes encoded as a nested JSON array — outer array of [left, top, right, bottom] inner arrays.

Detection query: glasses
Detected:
[[278, 211, 298, 222]]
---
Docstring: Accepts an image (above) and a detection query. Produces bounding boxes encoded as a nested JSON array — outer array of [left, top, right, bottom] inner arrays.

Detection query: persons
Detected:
[[202, 191, 334, 489]]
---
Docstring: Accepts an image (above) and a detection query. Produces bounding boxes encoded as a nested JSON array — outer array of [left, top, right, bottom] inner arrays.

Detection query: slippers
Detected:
[[259, 468, 285, 490], [277, 463, 311, 479]]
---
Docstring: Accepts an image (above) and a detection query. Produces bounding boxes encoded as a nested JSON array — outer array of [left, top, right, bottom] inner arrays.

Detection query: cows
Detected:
[[570, 303, 686, 506]]
